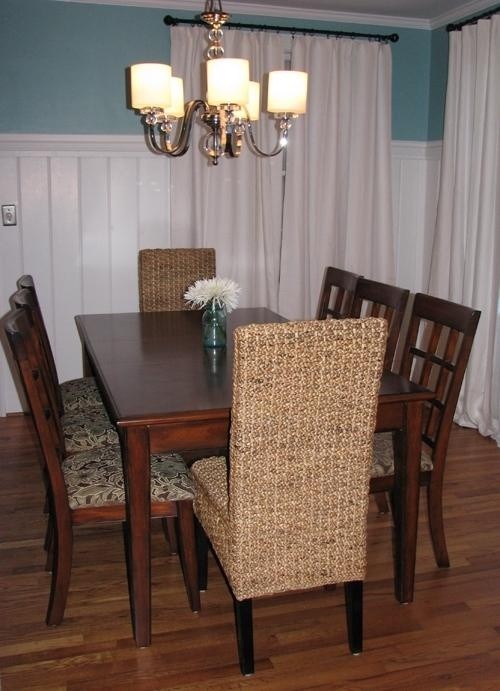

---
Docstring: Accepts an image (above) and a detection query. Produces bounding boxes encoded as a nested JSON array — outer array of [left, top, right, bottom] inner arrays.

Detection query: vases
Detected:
[[202, 303, 228, 348]]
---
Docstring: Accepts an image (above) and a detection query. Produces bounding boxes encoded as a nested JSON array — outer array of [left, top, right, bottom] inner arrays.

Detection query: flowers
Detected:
[[182, 275, 241, 316]]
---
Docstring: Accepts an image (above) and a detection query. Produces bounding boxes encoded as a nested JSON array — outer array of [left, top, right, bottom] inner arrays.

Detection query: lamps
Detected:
[[131, 1, 310, 165]]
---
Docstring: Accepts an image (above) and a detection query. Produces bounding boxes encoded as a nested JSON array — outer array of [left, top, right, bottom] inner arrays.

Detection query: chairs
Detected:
[[16, 274, 103, 412], [139, 247, 216, 312], [0, 310, 200, 628], [191, 318, 388, 679], [349, 276, 409, 514], [369, 292, 481, 568], [10, 289, 120, 510], [319, 265, 363, 320]]
